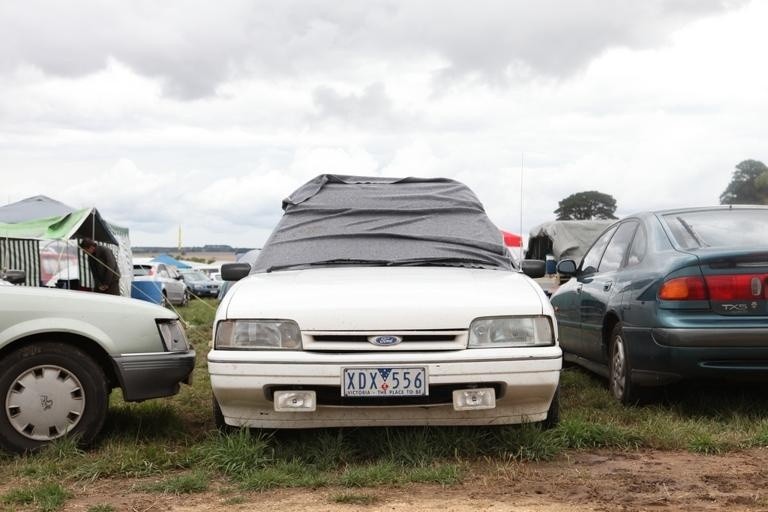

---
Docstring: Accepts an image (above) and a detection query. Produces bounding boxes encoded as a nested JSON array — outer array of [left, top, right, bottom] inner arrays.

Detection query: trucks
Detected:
[[522, 218, 620, 297]]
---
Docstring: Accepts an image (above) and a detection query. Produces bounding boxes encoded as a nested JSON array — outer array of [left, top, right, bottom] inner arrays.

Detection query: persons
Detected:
[[79, 238, 121, 296]]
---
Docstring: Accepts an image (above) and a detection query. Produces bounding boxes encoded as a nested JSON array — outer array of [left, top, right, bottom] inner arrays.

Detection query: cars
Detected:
[[548, 204, 768, 405], [205, 176, 562, 438], [0, 269, 197, 453], [32, 243, 234, 304]]
